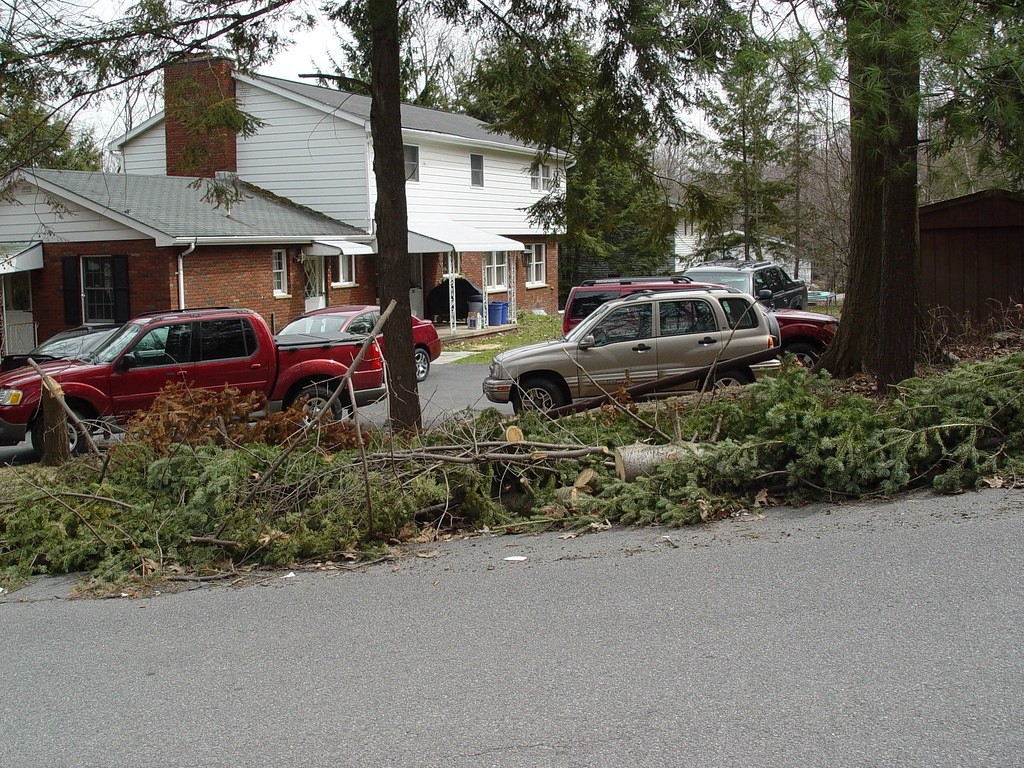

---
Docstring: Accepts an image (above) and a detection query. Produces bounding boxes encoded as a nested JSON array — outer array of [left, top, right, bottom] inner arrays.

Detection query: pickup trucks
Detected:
[[0, 306, 384, 460], [682, 260, 808, 312]]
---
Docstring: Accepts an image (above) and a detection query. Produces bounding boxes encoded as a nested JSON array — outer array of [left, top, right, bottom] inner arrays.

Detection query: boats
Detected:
[[808, 291, 837, 305]]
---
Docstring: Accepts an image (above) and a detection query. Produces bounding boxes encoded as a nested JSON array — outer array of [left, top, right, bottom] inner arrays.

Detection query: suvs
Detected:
[[563, 277, 840, 374], [482, 286, 781, 419]]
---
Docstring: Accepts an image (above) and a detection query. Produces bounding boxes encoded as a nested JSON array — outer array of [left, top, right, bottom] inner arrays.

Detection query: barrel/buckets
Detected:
[[488, 304, 502, 326], [492, 302, 508, 324], [469, 296, 482, 317], [468, 317, 476, 329]]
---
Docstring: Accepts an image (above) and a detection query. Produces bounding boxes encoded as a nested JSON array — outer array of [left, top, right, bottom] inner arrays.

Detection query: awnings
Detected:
[[313, 241, 375, 257], [406, 217, 526, 253]]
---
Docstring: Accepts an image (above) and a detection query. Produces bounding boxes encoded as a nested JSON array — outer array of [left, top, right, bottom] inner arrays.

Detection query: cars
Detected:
[[276, 305, 441, 396], [0, 321, 169, 385]]
